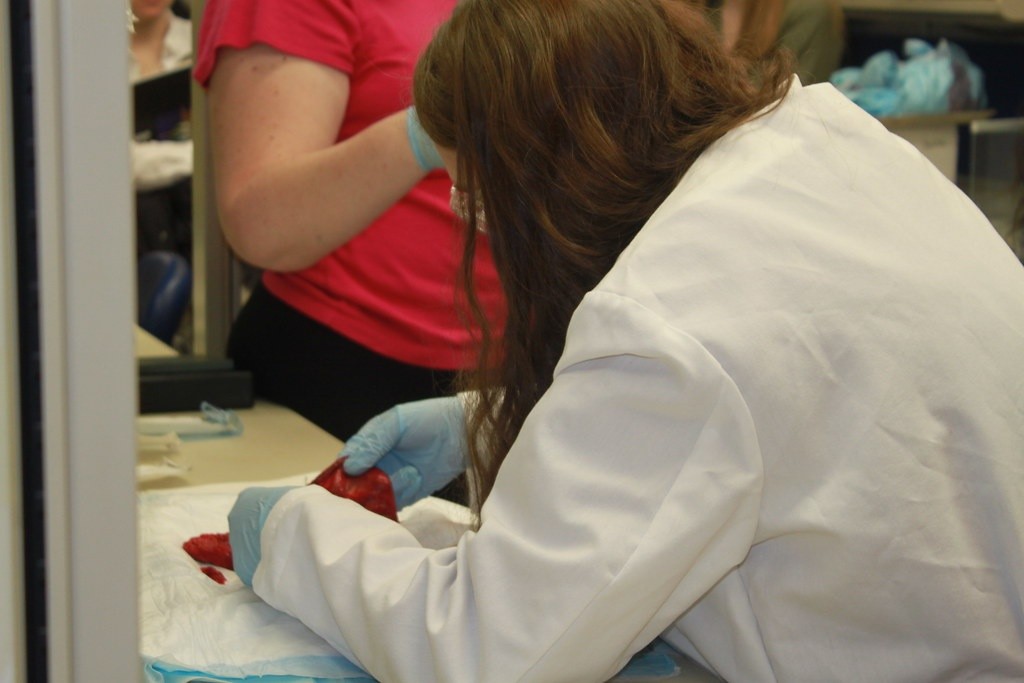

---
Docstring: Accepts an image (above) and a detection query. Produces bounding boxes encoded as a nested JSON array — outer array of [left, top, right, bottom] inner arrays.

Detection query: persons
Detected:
[[127, 0, 846, 505], [224, 0, 1024, 683]]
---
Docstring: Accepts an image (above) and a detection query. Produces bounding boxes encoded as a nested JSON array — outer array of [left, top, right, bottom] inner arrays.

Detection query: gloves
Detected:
[[407, 102, 446, 173], [227, 486, 293, 596], [335, 396, 472, 513]]
